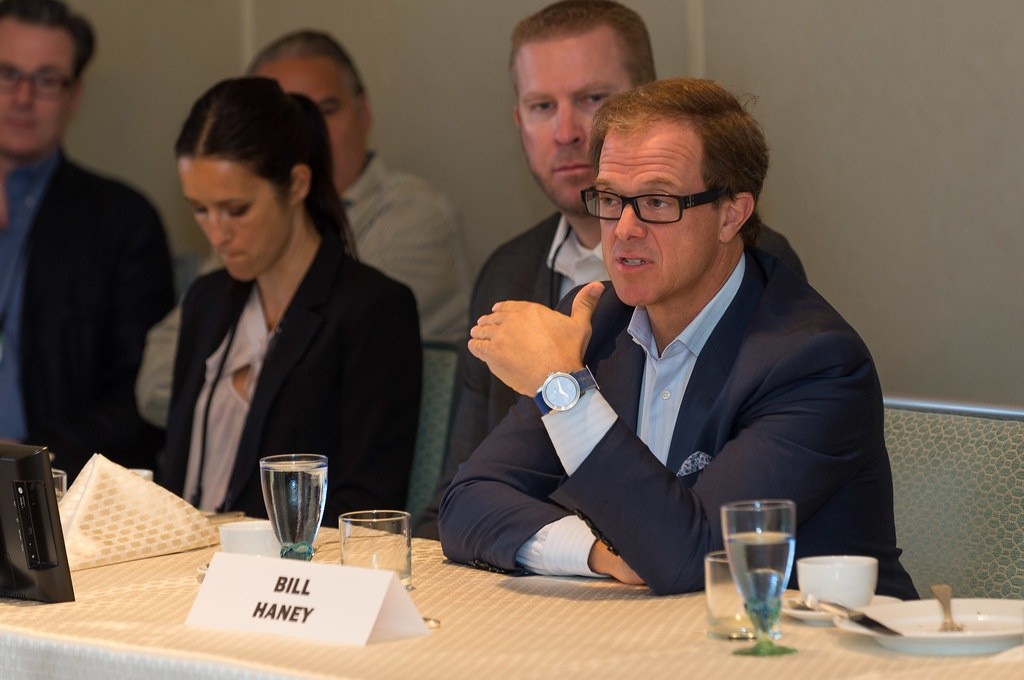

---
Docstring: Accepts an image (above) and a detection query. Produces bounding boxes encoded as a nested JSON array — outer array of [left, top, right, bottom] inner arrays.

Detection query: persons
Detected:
[[0, 0, 177, 493], [438, 78, 919, 602], [137, 28, 479, 425], [151, 76, 424, 533], [407, 0, 657, 541]]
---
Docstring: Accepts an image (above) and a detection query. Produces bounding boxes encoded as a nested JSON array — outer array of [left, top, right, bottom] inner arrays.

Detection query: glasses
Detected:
[[580, 186, 722, 224], [0, 61, 79, 102]]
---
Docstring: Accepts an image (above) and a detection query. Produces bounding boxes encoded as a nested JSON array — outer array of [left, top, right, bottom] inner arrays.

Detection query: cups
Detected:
[[218, 520, 281, 556], [51, 469, 67, 504], [797, 555, 878, 610], [339, 509, 411, 590], [704, 551, 781, 640], [129, 469, 153, 480]]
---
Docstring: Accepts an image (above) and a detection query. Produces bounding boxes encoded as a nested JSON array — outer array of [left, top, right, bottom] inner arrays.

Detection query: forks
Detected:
[[931, 585, 962, 632]]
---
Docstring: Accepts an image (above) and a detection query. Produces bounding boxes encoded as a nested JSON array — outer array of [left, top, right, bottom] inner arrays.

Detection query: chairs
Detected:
[[881, 394, 1024, 601], [399, 340, 460, 537]]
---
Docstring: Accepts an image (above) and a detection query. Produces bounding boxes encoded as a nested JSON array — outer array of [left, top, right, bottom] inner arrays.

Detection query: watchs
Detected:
[[534, 364, 600, 416]]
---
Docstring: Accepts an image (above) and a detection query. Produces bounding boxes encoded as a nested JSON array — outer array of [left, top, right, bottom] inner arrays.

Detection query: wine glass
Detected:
[[260, 454, 328, 561], [720, 499, 797, 657]]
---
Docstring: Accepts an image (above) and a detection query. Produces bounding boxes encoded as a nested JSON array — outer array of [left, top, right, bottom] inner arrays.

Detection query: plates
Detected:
[[782, 595, 903, 626], [833, 597, 1024, 655]]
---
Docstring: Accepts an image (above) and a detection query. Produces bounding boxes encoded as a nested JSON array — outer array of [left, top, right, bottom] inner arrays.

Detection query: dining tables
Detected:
[[1, 508, 1023, 678]]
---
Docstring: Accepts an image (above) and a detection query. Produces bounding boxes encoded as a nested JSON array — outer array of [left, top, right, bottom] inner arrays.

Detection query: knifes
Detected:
[[817, 601, 901, 637]]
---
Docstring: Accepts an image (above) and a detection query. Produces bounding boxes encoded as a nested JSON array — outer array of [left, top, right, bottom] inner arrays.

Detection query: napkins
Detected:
[[59, 453, 220, 573]]
